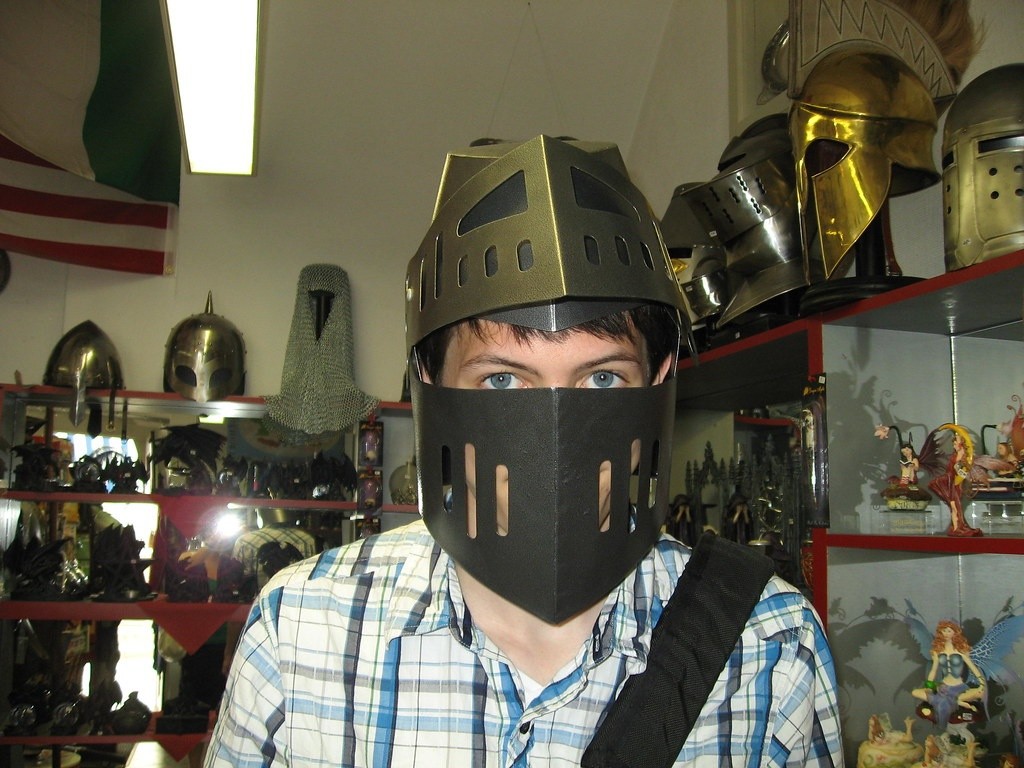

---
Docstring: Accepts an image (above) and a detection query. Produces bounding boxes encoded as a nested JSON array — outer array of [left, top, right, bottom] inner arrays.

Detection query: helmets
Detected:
[[161, 288, 245, 405], [662, 0, 1024, 333], [41, 317, 123, 426], [404, 135, 701, 356]]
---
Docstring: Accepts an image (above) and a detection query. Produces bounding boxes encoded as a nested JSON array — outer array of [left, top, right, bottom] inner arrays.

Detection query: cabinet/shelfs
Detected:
[[0, 255, 1024, 768]]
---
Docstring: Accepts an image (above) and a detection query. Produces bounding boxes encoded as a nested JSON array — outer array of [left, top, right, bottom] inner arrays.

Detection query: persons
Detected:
[[202, 133, 846, 768], [928, 432, 983, 536], [900, 442, 919, 487], [911, 618, 986, 712], [995, 438, 1017, 474]]
